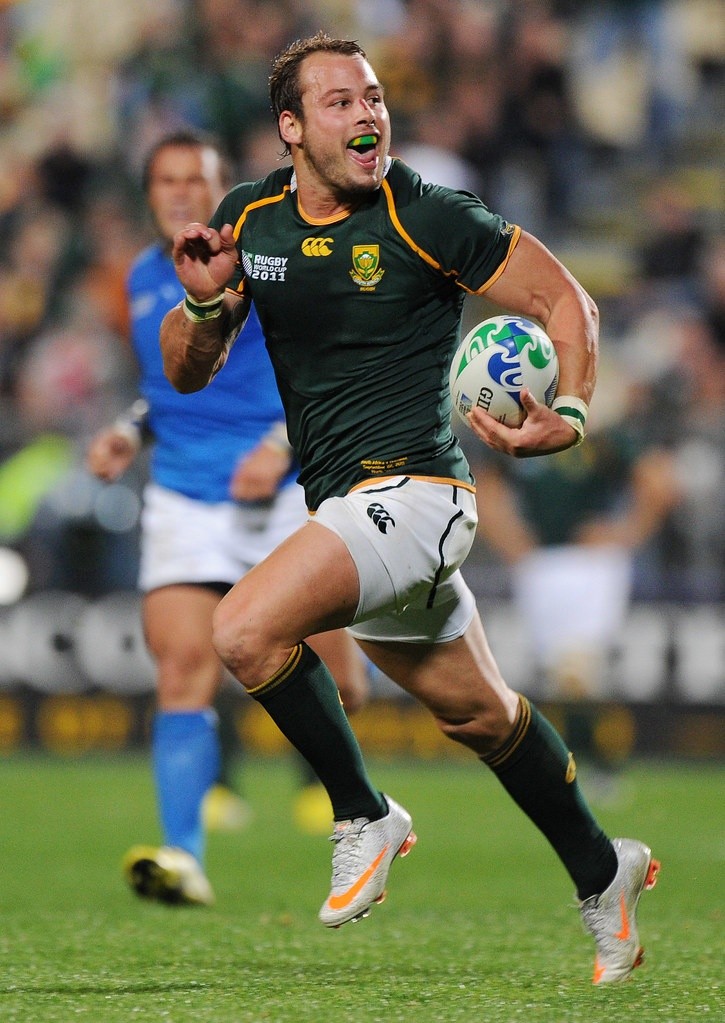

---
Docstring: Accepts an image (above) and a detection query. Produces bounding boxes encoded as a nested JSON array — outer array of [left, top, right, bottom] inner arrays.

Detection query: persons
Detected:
[[157, 34, 663, 988], [194, 677, 337, 836], [87, 116, 369, 904], [469, 364, 680, 810]]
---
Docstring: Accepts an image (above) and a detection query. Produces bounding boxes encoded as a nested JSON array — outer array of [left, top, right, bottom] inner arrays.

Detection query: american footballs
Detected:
[[448, 314, 560, 431]]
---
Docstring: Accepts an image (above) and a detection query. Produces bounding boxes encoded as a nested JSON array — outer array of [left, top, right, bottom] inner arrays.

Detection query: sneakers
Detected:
[[313, 792, 417, 929], [573, 837, 660, 985], [122, 846, 214, 909]]
